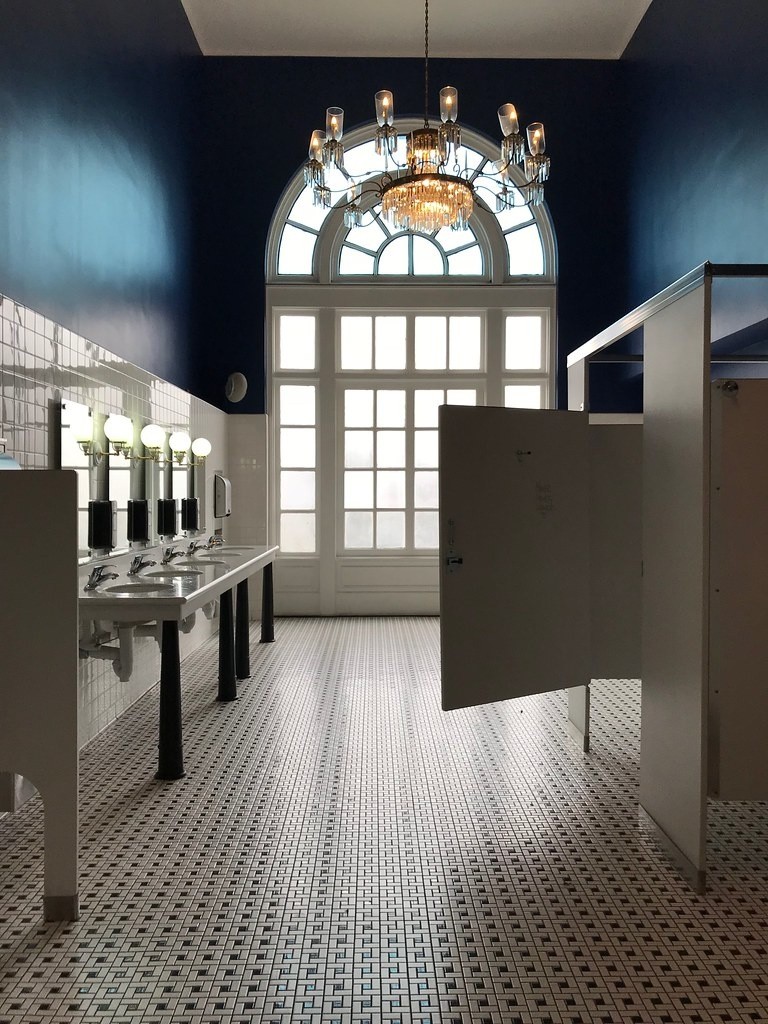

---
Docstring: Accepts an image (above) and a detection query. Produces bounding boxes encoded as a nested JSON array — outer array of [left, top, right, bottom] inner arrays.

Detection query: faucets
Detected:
[[160, 546, 185, 566], [83, 564, 120, 593], [185, 540, 210, 557], [126, 554, 158, 577], [206, 535, 226, 548]]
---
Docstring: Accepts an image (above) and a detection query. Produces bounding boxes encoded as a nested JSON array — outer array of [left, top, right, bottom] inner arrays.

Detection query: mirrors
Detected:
[[61, 398, 201, 565]]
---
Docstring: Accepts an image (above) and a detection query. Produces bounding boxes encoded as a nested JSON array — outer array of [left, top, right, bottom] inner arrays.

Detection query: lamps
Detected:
[[94, 414, 214, 466], [304, 0, 551, 232]]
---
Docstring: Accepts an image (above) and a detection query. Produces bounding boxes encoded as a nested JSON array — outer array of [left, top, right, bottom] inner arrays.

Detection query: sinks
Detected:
[[103, 581, 175, 595], [213, 546, 256, 552], [144, 569, 204, 580], [174, 559, 227, 568], [197, 551, 244, 560]]
